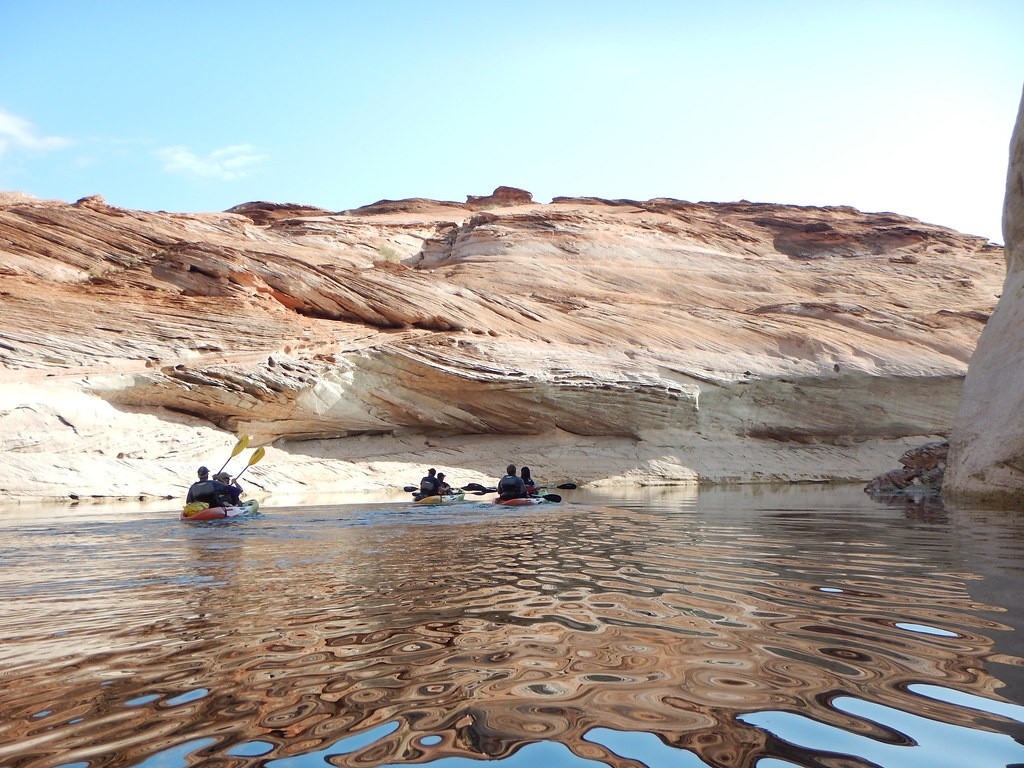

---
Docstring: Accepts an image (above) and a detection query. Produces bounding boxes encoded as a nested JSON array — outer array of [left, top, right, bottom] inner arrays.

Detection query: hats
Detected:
[[438, 473, 446, 477], [198, 466, 210, 475], [218, 472, 232, 478], [428, 469, 435, 475]]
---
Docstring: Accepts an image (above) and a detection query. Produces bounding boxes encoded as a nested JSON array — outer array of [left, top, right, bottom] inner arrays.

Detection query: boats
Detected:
[[493, 488, 549, 506], [414, 488, 465, 505], [180, 497, 261, 521]]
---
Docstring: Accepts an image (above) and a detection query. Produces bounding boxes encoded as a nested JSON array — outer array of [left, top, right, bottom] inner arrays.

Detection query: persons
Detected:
[[520, 466, 536, 495], [420, 468, 450, 497], [186, 466, 227, 508], [218, 472, 243, 506], [494, 464, 526, 497]]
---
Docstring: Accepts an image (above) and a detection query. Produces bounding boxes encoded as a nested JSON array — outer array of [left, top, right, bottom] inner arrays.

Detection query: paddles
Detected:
[[229, 446, 265, 488], [403, 482, 576, 504], [213, 434, 249, 481]]
